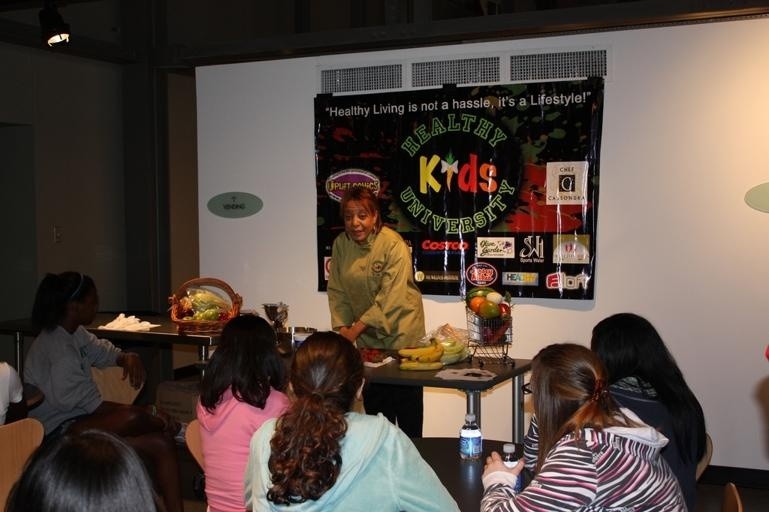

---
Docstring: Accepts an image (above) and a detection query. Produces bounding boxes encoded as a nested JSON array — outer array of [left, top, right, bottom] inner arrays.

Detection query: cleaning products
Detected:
[[464, 306, 516, 368]]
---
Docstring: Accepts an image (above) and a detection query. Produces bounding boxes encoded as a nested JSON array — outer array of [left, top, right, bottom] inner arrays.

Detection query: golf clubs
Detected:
[[465, 287, 496, 309]]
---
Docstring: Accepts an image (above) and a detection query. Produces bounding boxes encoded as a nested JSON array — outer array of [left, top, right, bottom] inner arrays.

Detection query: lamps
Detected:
[[691, 429, 714, 479], [0, 416, 46, 512], [88, 367, 147, 404], [720, 480, 744, 512]]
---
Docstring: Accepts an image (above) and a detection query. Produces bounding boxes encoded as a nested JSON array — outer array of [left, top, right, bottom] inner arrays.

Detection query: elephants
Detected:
[[359, 345, 385, 364]]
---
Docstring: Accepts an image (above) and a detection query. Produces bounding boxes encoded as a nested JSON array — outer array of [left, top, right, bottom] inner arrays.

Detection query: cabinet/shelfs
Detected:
[[459, 462, 482, 493], [458, 414, 482, 461], [500, 442, 524, 494]]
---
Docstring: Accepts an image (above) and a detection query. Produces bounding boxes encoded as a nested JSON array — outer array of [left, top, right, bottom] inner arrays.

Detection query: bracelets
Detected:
[[275, 326, 317, 348]]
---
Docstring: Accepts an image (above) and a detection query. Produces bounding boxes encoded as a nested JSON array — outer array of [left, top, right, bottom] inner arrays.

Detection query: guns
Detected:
[[397, 336, 444, 371], [438, 341, 470, 366]]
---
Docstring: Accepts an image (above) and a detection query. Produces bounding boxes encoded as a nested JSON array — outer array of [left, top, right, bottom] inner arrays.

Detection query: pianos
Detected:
[[488, 321, 510, 345]]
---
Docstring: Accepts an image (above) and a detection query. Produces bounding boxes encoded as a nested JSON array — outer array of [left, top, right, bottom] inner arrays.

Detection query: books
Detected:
[[154, 372, 203, 420]]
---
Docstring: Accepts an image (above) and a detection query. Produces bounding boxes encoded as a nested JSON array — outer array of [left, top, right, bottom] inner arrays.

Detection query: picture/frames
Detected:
[[267, 344, 534, 446], [401, 436, 535, 512], [1, 312, 222, 385]]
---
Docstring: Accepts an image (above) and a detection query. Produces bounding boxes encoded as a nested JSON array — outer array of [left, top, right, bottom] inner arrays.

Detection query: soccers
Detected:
[[478, 301, 499, 320], [497, 303, 511, 320], [181, 309, 219, 322]]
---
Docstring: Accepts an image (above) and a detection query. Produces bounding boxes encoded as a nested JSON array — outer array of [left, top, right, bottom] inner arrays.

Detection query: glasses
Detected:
[[36, 2, 73, 49]]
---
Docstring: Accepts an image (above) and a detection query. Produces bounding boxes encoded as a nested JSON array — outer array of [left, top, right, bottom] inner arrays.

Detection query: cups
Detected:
[[521, 383, 531, 395]]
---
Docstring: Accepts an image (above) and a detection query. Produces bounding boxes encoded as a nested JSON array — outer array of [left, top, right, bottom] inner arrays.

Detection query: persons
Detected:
[[194, 312, 290, 512], [326, 186, 427, 439], [243, 328, 463, 510], [478, 341, 689, 510], [0, 360, 30, 426], [3, 410, 184, 511], [588, 310, 707, 511], [23, 271, 186, 512]]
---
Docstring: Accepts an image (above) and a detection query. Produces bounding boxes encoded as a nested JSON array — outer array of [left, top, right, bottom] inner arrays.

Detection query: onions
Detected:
[[487, 291, 503, 305]]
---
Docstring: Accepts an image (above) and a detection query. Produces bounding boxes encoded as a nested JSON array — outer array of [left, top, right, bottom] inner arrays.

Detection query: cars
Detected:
[[292, 332, 313, 350]]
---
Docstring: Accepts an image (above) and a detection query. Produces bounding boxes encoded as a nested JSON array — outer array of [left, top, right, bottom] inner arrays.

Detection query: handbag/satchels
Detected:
[[149, 403, 158, 417]]
